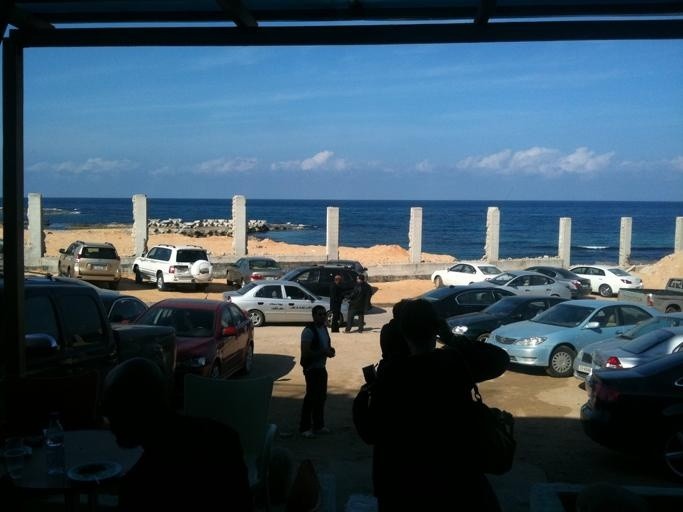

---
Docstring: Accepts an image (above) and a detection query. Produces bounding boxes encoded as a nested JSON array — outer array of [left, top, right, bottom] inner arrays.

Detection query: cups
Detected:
[[3, 447, 27, 478]]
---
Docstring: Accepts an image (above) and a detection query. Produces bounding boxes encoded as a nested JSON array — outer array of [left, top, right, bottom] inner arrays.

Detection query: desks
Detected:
[[0, 429, 143, 512]]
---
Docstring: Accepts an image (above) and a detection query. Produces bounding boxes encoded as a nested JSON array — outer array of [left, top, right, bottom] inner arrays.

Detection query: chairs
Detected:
[[480, 293, 491, 304], [272, 289, 280, 298], [279, 461, 323, 511], [182, 371, 273, 468]]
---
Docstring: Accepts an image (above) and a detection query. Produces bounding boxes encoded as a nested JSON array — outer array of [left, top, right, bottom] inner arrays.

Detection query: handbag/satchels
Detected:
[[473, 399, 514, 476]]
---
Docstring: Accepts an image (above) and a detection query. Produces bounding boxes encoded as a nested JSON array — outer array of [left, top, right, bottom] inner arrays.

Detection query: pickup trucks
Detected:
[[0, 275, 176, 434], [618, 277, 683, 312]]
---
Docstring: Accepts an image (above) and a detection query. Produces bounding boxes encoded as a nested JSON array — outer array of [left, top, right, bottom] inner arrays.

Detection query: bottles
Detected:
[[40, 415, 66, 471]]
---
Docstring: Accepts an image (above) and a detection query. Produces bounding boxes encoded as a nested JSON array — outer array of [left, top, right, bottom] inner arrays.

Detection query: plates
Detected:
[[64, 462, 123, 483]]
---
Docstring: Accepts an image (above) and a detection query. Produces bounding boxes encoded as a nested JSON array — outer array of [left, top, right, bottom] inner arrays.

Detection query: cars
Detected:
[[125, 297, 255, 387], [227, 256, 370, 306], [96, 288, 152, 328], [580, 348, 682, 473], [223, 279, 349, 327], [483, 299, 660, 378], [431, 262, 643, 297], [585, 325, 683, 402], [572, 310, 683, 380], [439, 296, 572, 345], [401, 284, 549, 334]]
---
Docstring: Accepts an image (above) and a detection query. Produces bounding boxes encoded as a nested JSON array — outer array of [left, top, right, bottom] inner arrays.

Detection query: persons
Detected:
[[97, 355, 252, 510], [349, 298, 513, 511], [376, 298, 411, 377], [329, 275, 344, 333], [297, 304, 336, 439], [342, 275, 372, 334]]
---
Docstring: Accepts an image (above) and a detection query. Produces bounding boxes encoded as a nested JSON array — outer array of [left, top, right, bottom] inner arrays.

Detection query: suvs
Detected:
[[58, 240, 121, 289], [131, 244, 215, 291]]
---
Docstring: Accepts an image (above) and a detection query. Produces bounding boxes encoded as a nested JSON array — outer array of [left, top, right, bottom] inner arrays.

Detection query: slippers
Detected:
[[296, 431, 316, 438], [312, 427, 330, 433]]
